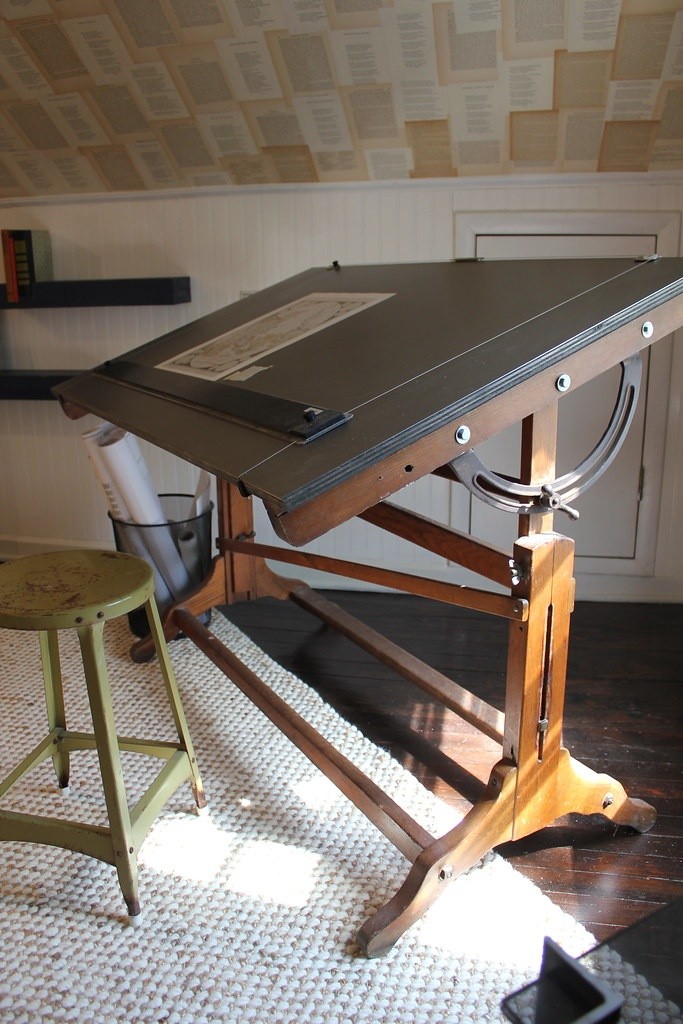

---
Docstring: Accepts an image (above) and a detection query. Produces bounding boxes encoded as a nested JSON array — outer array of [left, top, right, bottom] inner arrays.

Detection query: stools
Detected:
[[0, 551, 211, 919]]
